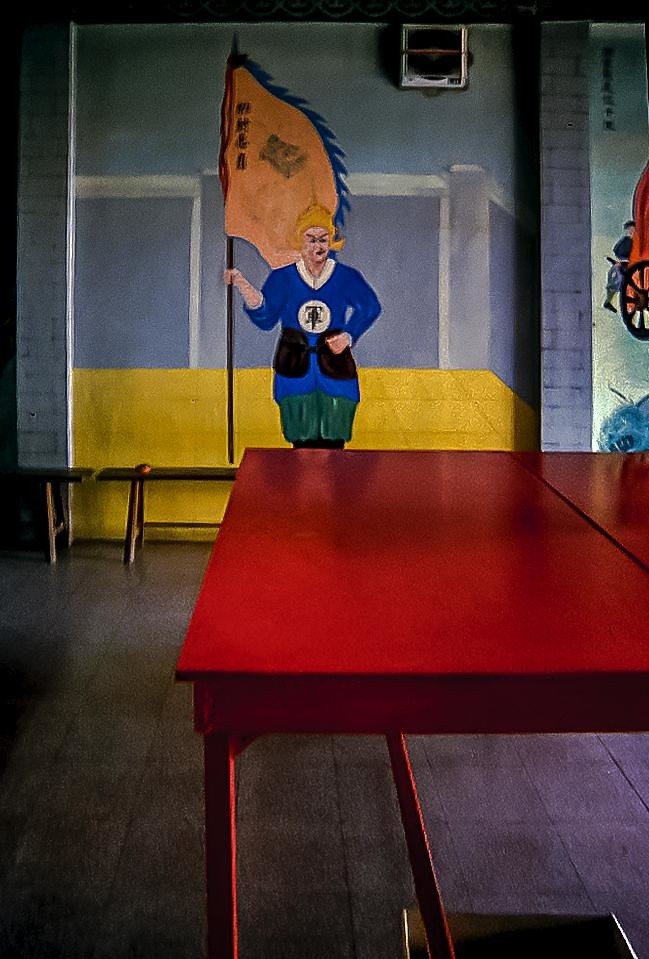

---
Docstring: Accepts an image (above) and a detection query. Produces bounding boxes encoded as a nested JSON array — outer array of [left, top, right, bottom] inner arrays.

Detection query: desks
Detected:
[[508, 450, 649, 572], [176, 445, 649, 959]]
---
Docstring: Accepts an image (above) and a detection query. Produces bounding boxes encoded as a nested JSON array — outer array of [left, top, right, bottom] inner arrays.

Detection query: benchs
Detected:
[[0, 469, 92, 565], [96, 467, 237, 566]]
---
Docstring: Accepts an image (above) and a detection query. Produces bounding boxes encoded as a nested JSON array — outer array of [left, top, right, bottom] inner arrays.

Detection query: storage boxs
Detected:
[[401, 906, 640, 959]]
